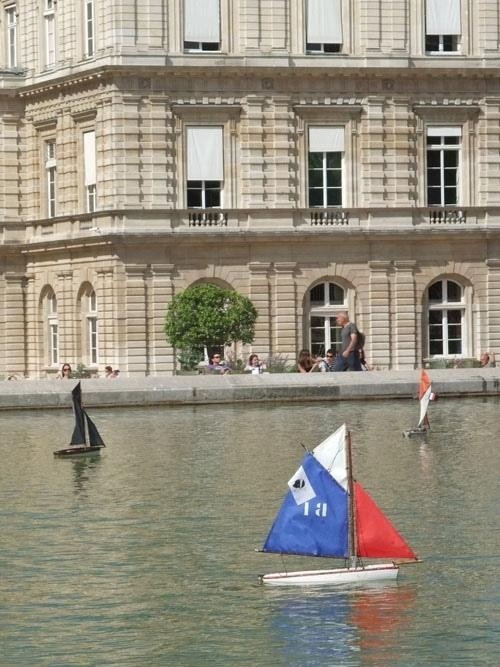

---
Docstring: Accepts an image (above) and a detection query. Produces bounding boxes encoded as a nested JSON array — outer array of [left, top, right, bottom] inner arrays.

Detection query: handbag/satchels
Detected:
[[356, 332, 365, 350]]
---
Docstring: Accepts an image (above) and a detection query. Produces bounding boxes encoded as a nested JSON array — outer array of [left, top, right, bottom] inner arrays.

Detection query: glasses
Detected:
[[326, 354, 333, 358]]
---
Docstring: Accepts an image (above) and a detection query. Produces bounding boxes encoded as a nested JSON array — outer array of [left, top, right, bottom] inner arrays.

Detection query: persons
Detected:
[[480, 349, 496, 368], [103, 365, 119, 378], [60, 362, 73, 379], [244, 353, 271, 375], [317, 348, 339, 372], [205, 352, 232, 374], [296, 347, 322, 371], [331, 309, 361, 371]]
